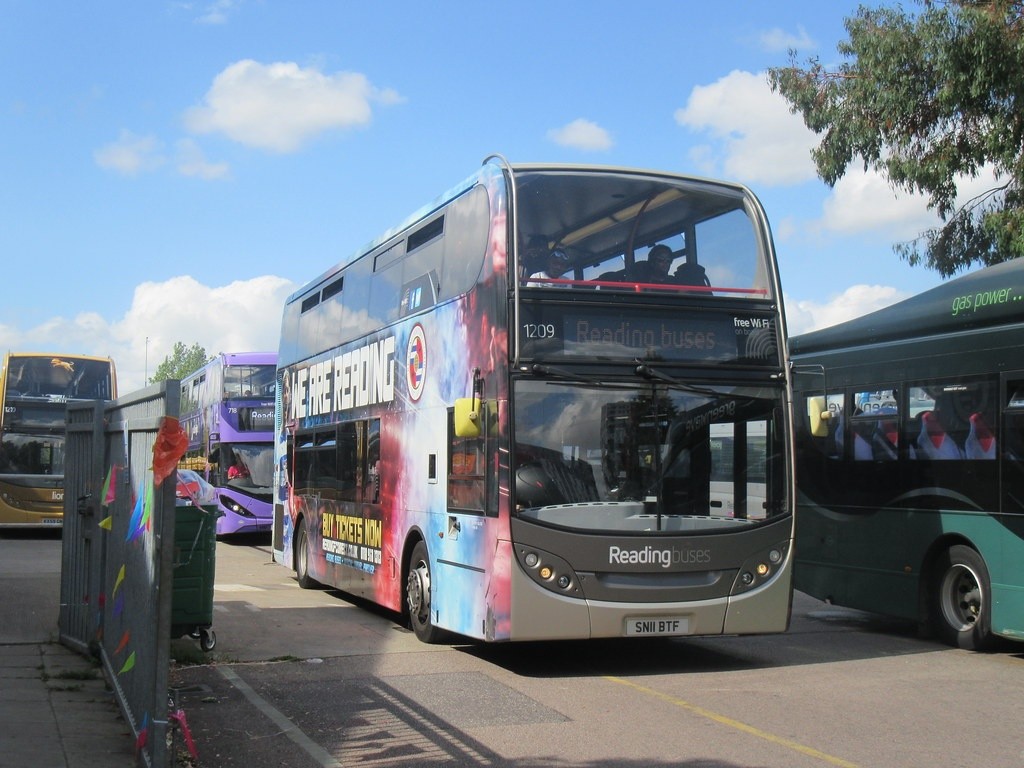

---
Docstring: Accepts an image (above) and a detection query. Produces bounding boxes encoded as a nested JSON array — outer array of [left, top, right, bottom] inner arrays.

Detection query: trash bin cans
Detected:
[[171, 468, 217, 652]]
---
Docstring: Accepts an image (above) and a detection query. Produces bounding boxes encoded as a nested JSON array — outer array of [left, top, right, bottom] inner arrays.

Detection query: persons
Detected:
[[227, 454, 250, 480], [643, 245, 680, 285], [526, 249, 573, 288], [244, 390, 253, 397]]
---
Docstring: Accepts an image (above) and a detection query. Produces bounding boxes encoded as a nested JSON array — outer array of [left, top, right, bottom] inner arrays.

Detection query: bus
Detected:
[[175, 350, 280, 538], [270, 153, 797, 644], [0, 349, 119, 531], [790, 253, 1024, 655]]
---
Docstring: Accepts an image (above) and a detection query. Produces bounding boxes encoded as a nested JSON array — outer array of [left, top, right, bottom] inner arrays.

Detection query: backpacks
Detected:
[[675, 264, 713, 297]]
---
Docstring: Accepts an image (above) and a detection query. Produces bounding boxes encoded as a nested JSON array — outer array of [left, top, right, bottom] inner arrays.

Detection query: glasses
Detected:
[[549, 250, 570, 261], [652, 254, 673, 265]]
